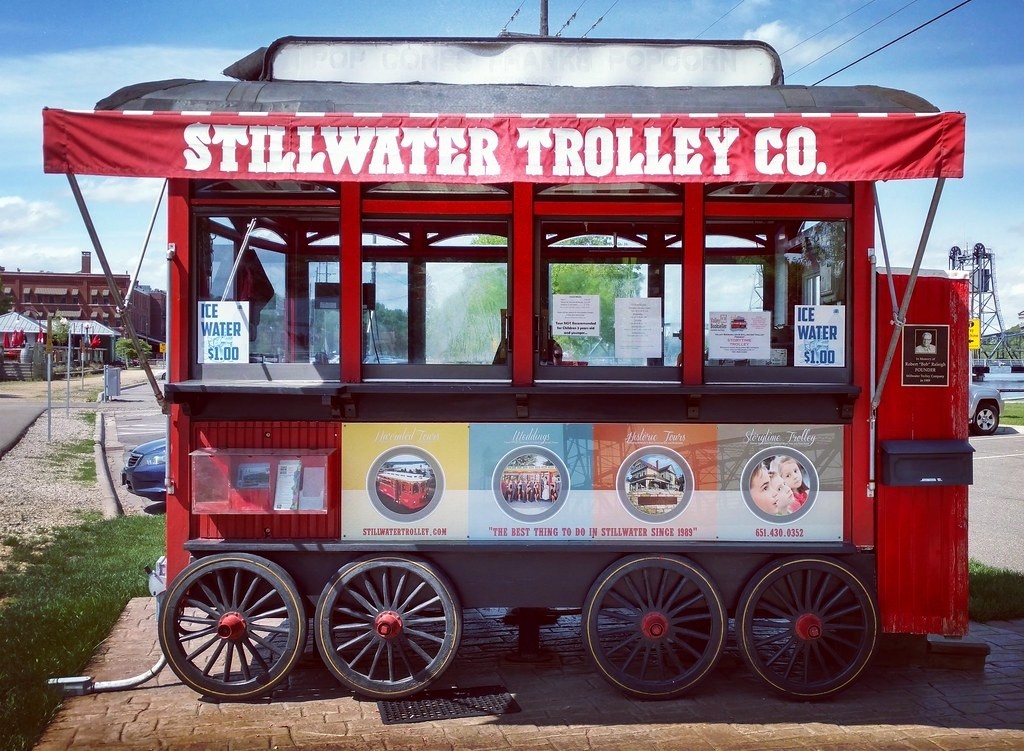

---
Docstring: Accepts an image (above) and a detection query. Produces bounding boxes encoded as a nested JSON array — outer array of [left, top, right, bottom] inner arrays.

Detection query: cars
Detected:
[[121, 436, 166, 501], [249, 353, 284, 363], [111, 359, 139, 369], [238, 468, 271, 489], [329, 354, 409, 364], [154, 369, 166, 380], [969, 381, 1004, 435]]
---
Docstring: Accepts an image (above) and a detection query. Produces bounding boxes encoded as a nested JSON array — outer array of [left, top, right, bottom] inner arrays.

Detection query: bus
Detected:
[[502, 464, 560, 497], [377, 471, 433, 509]]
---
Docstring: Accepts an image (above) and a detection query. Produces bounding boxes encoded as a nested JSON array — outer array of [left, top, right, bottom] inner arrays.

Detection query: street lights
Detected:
[[145, 322, 148, 343], [59, 317, 71, 418], [84, 323, 91, 364]]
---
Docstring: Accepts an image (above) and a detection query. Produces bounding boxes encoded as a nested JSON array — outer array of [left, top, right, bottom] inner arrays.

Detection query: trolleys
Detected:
[[45, 36, 972, 702]]
